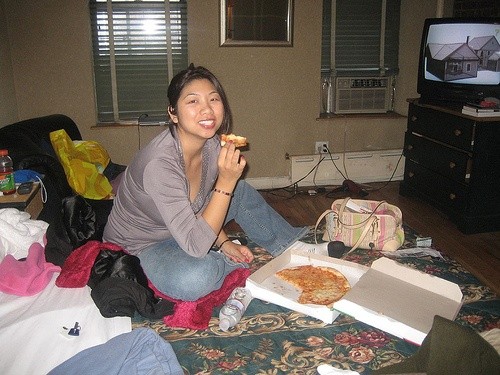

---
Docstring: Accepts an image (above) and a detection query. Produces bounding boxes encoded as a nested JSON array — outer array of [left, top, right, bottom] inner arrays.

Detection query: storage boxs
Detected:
[[246, 241, 465, 346]]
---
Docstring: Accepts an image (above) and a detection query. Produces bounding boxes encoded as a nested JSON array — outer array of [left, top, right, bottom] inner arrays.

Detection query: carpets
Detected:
[[130, 224, 500, 375]]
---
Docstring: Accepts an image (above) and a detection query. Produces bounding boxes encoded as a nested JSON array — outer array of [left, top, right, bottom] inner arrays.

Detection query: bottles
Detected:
[[219, 287, 251, 331], [0, 150, 16, 195]]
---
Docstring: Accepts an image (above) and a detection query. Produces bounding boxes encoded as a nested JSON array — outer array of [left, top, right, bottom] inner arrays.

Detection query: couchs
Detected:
[[0, 116, 129, 250]]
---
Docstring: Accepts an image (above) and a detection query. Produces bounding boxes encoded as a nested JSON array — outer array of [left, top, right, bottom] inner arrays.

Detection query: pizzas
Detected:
[[274, 266, 351, 305], [220, 133, 247, 147]]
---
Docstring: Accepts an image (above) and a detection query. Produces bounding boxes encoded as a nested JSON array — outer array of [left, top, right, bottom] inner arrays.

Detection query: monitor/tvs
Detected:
[[416, 18, 500, 107]]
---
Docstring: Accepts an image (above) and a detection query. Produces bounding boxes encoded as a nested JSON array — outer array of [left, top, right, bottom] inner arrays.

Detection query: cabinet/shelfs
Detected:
[[397, 99, 500, 233]]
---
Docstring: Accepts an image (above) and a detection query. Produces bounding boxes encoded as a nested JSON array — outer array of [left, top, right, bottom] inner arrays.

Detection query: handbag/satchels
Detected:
[[314, 197, 405, 258]]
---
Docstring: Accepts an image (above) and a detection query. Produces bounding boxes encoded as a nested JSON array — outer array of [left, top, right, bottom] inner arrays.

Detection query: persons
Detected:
[[102, 66, 345, 302]]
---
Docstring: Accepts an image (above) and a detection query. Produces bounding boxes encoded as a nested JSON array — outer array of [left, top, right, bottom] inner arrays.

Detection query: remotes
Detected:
[[17, 182, 33, 195]]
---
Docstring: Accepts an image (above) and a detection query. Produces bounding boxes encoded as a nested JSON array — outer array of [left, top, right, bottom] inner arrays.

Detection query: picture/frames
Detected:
[[218, 0, 294, 47]]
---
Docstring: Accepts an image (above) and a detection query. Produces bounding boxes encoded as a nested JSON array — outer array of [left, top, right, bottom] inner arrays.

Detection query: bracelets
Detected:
[[220, 240, 230, 248], [214, 189, 234, 196]]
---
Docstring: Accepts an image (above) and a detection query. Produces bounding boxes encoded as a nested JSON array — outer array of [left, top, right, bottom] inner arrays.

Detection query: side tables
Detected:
[[0, 180, 44, 220]]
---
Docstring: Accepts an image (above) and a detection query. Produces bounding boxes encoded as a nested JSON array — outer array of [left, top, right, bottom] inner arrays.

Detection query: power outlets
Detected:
[[315, 142, 329, 154]]
